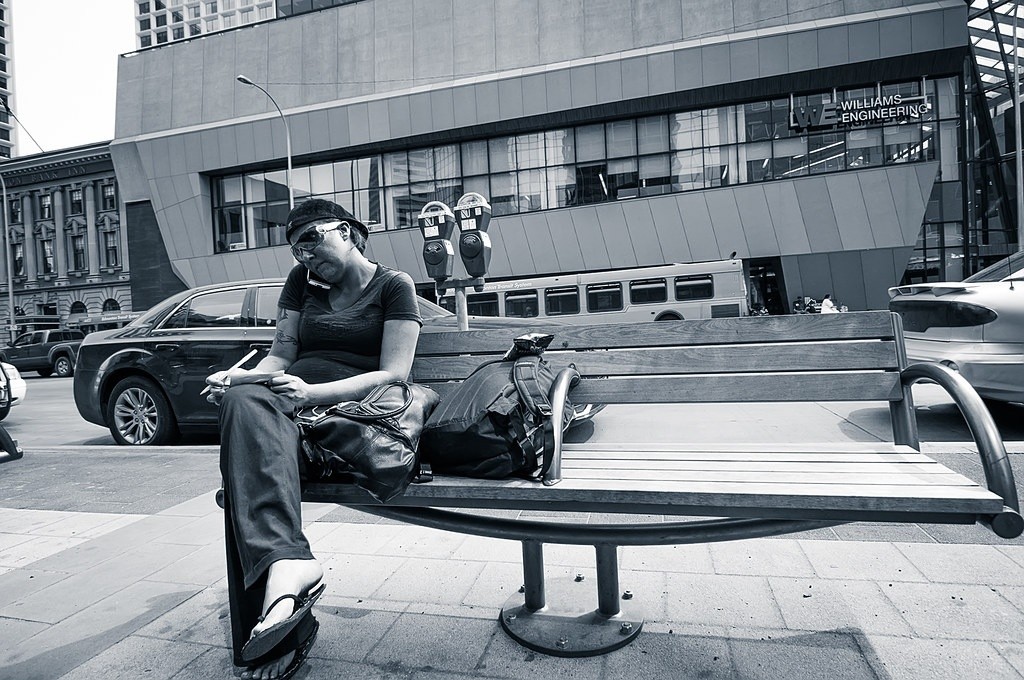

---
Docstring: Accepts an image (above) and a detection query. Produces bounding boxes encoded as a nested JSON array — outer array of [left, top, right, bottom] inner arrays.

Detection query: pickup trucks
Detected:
[[0, 326, 85, 374]]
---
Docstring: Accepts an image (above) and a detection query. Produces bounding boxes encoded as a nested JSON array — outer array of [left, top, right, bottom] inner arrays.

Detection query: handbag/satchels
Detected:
[[293, 380, 440, 504]]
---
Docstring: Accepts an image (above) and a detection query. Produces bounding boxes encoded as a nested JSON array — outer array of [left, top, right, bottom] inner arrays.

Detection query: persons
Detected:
[[793, 296, 804, 313], [821, 294, 834, 313], [206, 198, 424, 680]]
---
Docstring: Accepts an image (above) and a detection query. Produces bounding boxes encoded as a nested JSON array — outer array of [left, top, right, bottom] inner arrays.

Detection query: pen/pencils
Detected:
[[199, 347, 257, 400]]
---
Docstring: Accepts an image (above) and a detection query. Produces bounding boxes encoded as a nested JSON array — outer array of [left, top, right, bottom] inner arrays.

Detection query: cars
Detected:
[[0, 361, 27, 408], [70, 277, 606, 448], [47, 338, 84, 377], [887, 245, 1024, 405]]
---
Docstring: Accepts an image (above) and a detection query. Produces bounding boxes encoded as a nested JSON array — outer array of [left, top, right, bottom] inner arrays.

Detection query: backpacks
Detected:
[[421, 355, 577, 479]]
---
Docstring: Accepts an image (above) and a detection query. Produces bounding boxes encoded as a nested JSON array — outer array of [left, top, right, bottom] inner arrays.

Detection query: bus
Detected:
[[429, 250, 753, 325]]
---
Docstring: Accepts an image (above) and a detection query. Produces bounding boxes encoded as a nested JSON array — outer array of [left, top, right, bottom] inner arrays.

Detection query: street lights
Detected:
[[236, 73, 295, 214]]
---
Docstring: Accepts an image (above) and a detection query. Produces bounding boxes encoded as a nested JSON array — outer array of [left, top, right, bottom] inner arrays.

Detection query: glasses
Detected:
[[290, 222, 343, 262]]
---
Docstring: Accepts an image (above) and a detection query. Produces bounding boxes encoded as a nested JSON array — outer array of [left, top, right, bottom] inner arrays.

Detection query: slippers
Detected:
[[278, 620, 320, 680], [240, 583, 327, 663]]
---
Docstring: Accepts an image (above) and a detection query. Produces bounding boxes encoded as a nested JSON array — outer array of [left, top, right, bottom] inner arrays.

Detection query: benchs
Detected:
[[215, 311, 1023, 679]]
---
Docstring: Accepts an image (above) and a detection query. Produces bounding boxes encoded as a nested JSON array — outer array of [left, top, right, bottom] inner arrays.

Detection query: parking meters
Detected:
[[415, 192, 493, 333]]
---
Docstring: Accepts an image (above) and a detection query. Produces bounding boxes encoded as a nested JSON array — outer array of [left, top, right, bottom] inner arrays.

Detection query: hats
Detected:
[[797, 296, 802, 300], [286, 199, 368, 245]]
